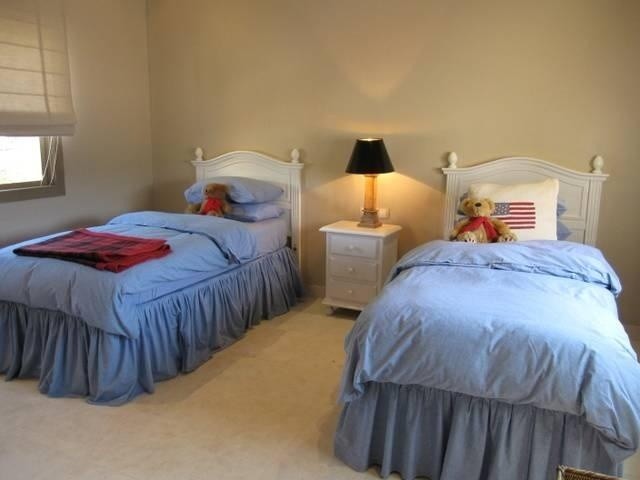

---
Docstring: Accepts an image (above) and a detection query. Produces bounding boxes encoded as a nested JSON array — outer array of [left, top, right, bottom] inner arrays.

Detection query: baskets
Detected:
[[556, 464, 624, 480]]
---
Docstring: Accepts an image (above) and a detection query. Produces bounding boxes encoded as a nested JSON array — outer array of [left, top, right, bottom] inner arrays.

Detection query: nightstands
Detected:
[[318, 219, 403, 313]]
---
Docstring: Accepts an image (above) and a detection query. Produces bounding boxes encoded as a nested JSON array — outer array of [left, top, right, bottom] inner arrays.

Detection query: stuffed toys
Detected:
[[448, 197, 517, 244], [187, 182, 233, 217]]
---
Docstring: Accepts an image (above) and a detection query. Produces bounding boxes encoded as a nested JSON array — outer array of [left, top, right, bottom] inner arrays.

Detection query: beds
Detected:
[[0, 144, 305, 405], [364, 152, 640, 480]]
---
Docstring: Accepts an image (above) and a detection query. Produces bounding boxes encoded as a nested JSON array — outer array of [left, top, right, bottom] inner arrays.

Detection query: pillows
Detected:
[[467, 177, 560, 241], [222, 201, 285, 222], [184, 177, 284, 204]]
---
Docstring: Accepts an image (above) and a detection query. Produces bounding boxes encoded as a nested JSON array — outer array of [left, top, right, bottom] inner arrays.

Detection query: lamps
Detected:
[[346, 137, 395, 228]]
